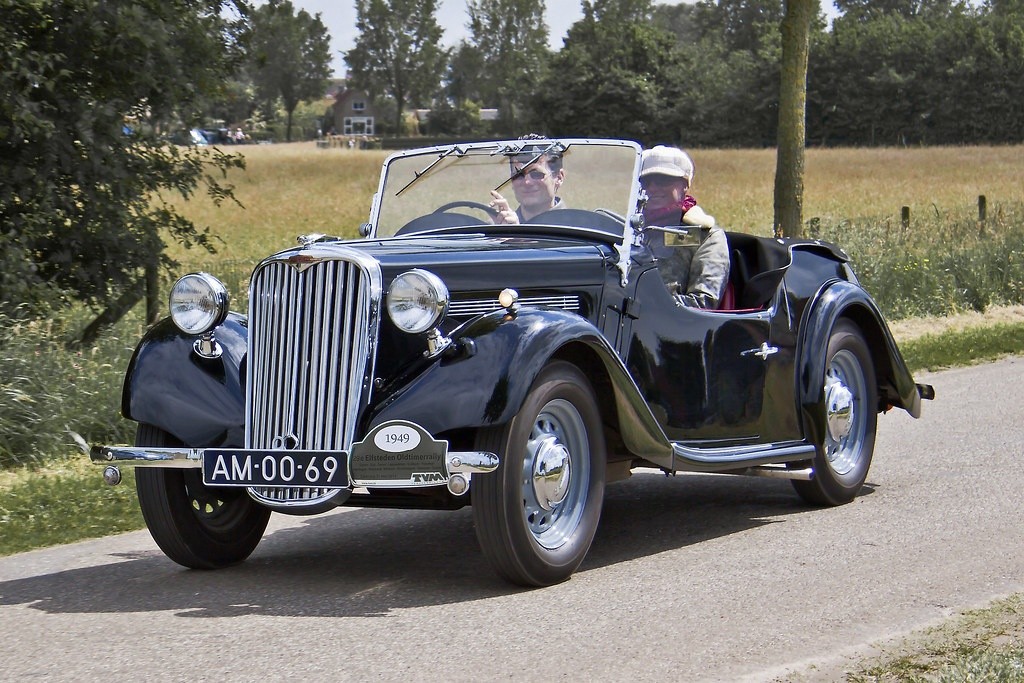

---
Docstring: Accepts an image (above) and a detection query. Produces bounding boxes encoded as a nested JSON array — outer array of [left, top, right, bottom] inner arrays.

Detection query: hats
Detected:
[[638, 145, 692, 190]]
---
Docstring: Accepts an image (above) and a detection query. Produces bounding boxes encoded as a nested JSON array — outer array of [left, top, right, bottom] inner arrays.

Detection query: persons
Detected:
[[234, 128, 244, 144], [490, 133, 565, 224], [640, 144, 730, 310]]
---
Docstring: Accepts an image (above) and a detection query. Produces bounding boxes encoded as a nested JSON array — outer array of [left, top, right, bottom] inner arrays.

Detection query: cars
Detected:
[[86, 135, 935, 584], [172, 128, 239, 147]]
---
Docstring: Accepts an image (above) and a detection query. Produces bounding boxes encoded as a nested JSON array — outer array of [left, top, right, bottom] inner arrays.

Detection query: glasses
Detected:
[[640, 176, 685, 190], [512, 171, 557, 183]]
